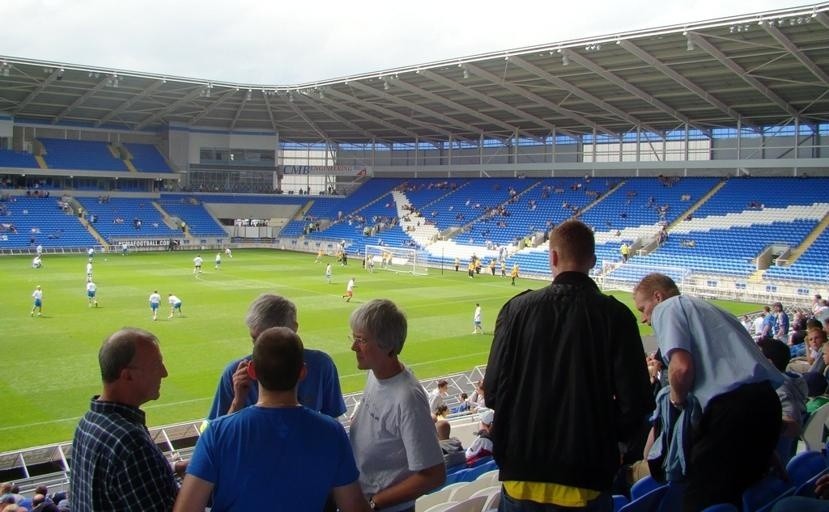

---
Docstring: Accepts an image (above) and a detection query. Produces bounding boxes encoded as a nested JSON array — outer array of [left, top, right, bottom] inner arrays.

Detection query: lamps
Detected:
[[44, 66, 67, 79], [413, 69, 425, 78], [367, 73, 399, 92], [195, 83, 214, 99], [727, 12, 829, 33], [682, 30, 696, 53], [344, 82, 349, 87], [539, 40, 623, 66], [2, 61, 17, 77], [160, 80, 168, 86], [235, 86, 327, 105], [88, 72, 126, 90], [457, 63, 472, 80], [504, 56, 511, 61]]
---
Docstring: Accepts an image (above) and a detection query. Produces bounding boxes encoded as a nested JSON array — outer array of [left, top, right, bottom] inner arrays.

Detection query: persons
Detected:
[[193, 255, 203, 273], [87, 247, 98, 308], [0, 176, 142, 248], [303, 180, 457, 247], [150, 290, 161, 321], [224, 246, 233, 258], [167, 293, 184, 319], [32, 244, 42, 268], [161, 181, 339, 195], [215, 253, 222, 269], [615, 242, 829, 512], [454, 254, 520, 287], [455, 177, 694, 247], [31, 285, 43, 317], [747, 198, 765, 212], [314, 244, 416, 303], [428, 377, 496, 465], [2, 293, 446, 512], [482, 221, 655, 512], [472, 303, 484, 334]]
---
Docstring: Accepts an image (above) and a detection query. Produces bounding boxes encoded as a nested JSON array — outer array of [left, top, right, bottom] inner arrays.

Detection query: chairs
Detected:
[[2, 133, 231, 249], [15, 338, 828, 511], [276, 169, 829, 309], [161, 187, 313, 239]]
